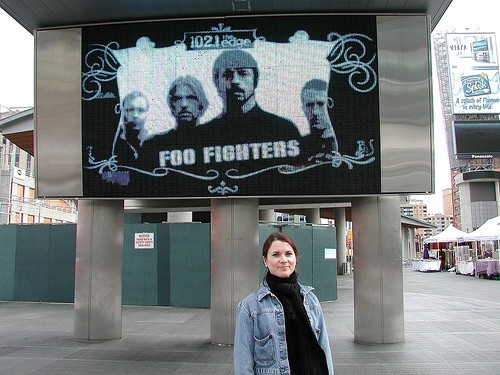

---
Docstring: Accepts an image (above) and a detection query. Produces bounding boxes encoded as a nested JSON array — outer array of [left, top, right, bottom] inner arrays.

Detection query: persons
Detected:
[[233, 231, 334, 375], [122, 50, 336, 174], [437, 248, 445, 271], [424, 248, 429, 260]]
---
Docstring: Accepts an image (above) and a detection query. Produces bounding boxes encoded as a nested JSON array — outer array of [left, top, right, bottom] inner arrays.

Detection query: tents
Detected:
[[424, 215, 500, 280]]
